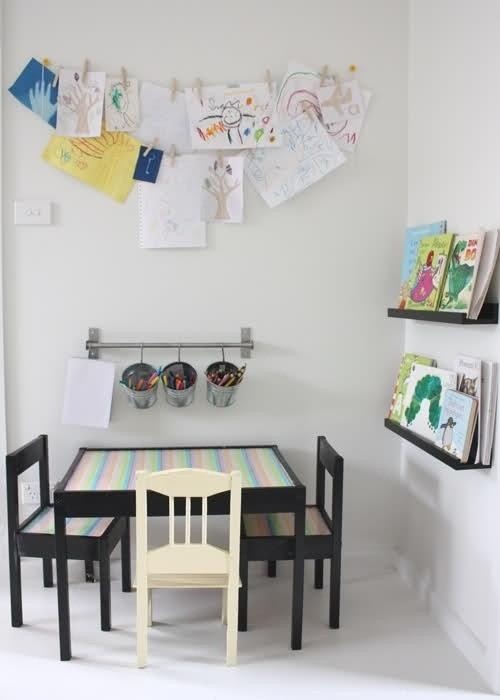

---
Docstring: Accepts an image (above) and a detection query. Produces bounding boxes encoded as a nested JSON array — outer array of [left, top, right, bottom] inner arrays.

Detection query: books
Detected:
[[389, 217, 500, 324], [386, 344, 500, 469]]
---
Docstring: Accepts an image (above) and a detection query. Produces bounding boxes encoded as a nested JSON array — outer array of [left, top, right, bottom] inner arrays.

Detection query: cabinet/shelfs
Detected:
[[384, 304, 499, 471]]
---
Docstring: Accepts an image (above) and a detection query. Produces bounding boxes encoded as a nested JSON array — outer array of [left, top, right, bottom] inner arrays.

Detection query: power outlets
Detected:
[[21, 483, 41, 506]]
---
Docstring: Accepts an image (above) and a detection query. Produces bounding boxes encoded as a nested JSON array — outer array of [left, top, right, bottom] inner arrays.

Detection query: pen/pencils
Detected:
[[161, 370, 197, 390], [120, 366, 162, 391], [204, 366, 246, 386]]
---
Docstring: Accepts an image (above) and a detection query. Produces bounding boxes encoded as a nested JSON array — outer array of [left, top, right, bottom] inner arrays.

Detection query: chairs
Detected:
[[6, 435, 130, 630], [134, 467, 242, 668], [239, 435, 343, 632]]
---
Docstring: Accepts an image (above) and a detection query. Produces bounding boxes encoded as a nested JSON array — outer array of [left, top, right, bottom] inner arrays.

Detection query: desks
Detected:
[[54, 446, 307, 661]]
